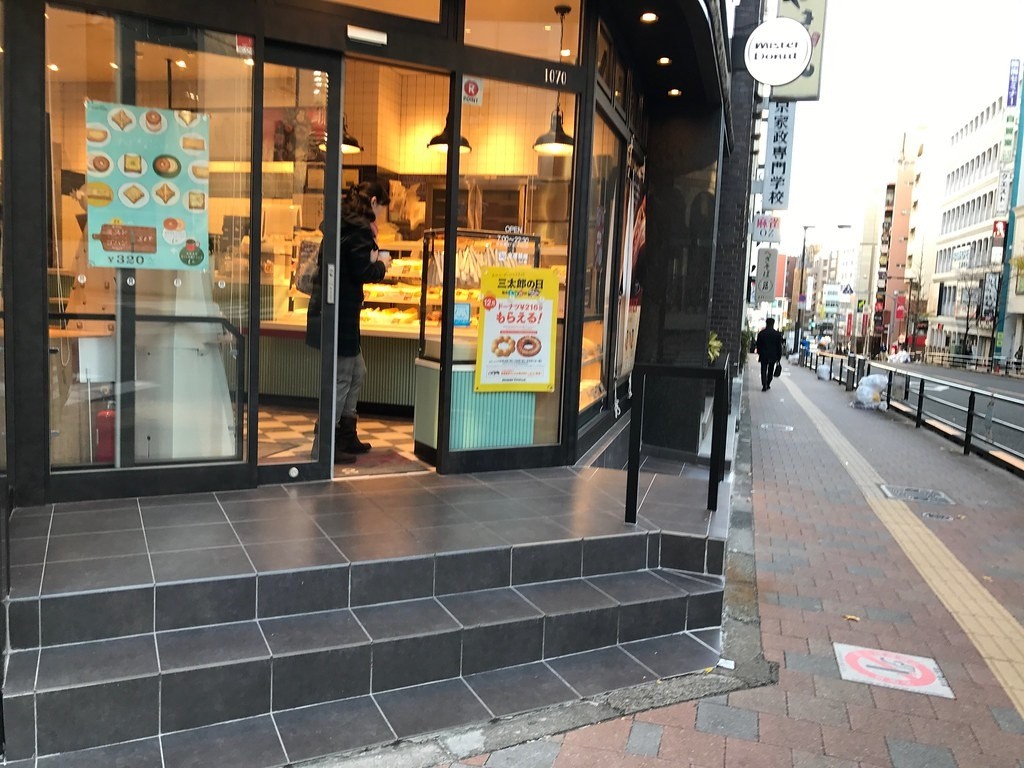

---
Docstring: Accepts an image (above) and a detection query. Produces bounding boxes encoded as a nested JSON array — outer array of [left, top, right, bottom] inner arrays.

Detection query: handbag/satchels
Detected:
[[296, 247, 320, 296], [773, 360, 782, 377]]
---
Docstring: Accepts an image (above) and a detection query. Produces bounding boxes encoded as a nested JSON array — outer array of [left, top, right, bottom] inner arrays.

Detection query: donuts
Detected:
[[492, 336, 542, 356]]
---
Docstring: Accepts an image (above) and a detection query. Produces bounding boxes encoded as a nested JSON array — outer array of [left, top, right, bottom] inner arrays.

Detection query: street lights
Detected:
[[876, 290, 898, 355], [794, 224, 851, 353], [884, 276, 914, 352]]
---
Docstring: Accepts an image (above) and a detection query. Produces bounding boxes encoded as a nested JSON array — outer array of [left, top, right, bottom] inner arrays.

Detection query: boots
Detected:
[[336, 414, 372, 454], [310, 420, 357, 464]]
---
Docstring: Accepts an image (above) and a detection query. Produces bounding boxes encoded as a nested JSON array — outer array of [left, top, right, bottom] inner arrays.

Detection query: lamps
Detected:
[[531, 4, 576, 158], [427, 110, 472, 155], [318, 109, 362, 155]]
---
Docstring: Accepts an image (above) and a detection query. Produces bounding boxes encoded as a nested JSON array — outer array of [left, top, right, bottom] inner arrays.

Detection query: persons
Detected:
[[1014, 345, 1023, 375], [757, 318, 782, 392], [305, 180, 392, 462]]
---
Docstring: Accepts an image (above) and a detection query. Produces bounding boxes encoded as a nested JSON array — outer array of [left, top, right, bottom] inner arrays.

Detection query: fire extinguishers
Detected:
[[89, 385, 115, 463]]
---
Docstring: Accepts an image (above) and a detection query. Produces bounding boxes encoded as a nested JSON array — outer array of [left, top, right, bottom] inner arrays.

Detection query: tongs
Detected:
[[427, 245, 516, 289]]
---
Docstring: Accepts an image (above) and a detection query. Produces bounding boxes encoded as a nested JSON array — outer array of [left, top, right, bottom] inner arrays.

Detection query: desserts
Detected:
[[87, 110, 208, 242]]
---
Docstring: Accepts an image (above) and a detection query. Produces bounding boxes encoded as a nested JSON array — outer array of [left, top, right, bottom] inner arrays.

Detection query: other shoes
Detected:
[[762, 388, 766, 392], [766, 386, 770, 389]]
